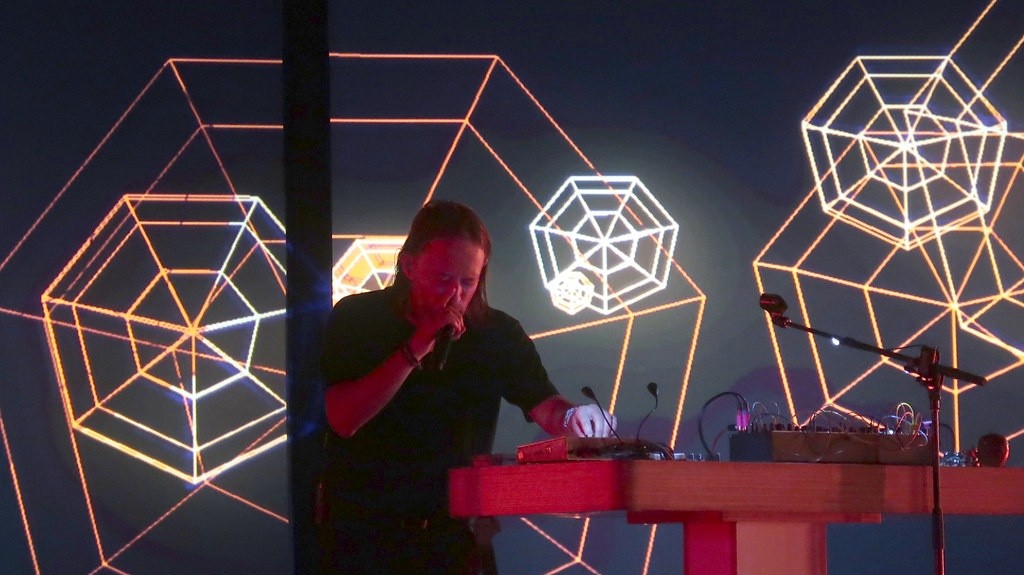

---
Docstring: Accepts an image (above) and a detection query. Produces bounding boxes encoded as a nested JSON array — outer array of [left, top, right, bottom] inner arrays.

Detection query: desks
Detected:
[[450, 460, 1024, 575]]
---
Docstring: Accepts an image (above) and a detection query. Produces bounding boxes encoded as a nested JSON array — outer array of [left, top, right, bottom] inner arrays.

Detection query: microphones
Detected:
[[433, 327, 455, 371]]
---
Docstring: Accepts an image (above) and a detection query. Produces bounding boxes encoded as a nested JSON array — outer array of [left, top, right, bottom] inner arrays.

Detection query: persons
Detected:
[[315, 202, 618, 574]]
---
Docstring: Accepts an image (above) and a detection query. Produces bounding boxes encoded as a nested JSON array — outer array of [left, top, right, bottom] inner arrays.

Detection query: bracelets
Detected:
[[563, 405, 579, 430], [400, 342, 422, 373]]
